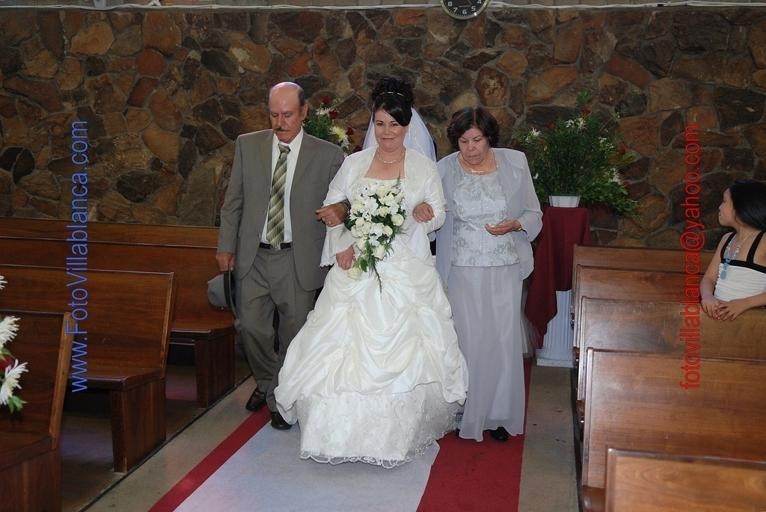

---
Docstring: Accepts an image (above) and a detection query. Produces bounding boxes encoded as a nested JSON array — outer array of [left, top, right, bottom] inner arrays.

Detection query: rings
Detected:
[[329, 221, 331, 225]]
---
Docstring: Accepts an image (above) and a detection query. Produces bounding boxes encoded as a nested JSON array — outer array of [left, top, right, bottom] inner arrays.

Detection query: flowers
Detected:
[[514, 86, 644, 226], [348, 170, 408, 295]]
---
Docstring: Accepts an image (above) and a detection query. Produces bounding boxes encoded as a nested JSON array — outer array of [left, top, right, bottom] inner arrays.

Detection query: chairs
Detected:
[[0, 216, 235, 512], [573, 245, 766, 512]]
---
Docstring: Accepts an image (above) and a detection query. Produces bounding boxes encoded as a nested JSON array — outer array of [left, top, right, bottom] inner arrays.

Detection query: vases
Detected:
[[548, 195, 580, 208]]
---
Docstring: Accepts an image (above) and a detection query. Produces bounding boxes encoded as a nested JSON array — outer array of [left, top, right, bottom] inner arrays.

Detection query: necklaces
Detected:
[[719, 232, 750, 279], [376, 153, 403, 164]]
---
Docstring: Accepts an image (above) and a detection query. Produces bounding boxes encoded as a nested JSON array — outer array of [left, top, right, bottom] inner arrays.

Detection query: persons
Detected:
[[412, 105, 544, 442], [700, 184, 766, 321], [216, 81, 351, 430], [273, 76, 470, 469]]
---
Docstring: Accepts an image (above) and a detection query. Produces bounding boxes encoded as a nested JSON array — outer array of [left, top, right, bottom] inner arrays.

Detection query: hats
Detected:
[[207, 262, 240, 320]]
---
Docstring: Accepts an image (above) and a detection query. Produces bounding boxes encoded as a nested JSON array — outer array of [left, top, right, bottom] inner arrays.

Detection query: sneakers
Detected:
[[270, 411, 292, 430], [245, 387, 267, 412], [490, 427, 510, 442]]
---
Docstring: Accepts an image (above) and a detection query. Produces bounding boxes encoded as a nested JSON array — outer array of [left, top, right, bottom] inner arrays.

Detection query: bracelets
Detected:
[[516, 226, 521, 232]]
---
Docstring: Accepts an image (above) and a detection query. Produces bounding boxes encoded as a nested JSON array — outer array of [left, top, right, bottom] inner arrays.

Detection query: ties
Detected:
[[266, 143, 291, 251]]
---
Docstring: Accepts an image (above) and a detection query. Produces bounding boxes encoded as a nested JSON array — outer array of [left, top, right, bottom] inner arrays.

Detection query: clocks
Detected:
[[441, 0, 489, 20]]
